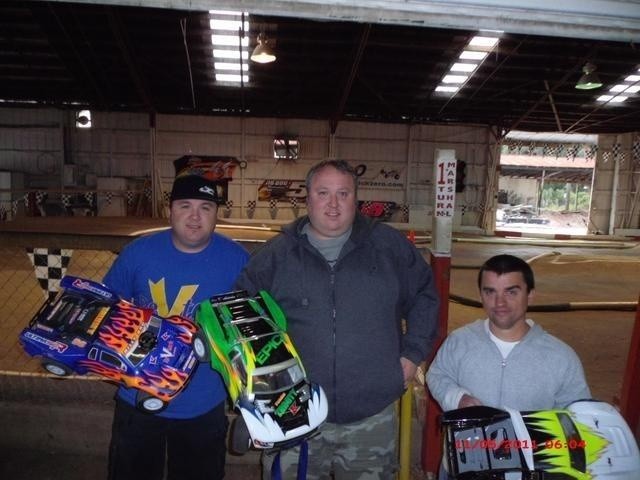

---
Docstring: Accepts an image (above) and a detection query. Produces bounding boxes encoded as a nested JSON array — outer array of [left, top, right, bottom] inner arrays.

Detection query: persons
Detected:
[[102, 171, 257, 480], [424, 254, 591, 480], [233, 159, 442, 480]]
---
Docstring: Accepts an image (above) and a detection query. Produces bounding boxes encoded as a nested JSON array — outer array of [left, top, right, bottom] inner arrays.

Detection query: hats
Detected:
[[170, 175, 218, 203]]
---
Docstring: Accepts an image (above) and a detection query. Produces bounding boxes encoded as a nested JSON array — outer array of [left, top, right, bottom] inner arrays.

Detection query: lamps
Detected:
[[250, 32, 276, 64], [574, 63, 603, 90]]
